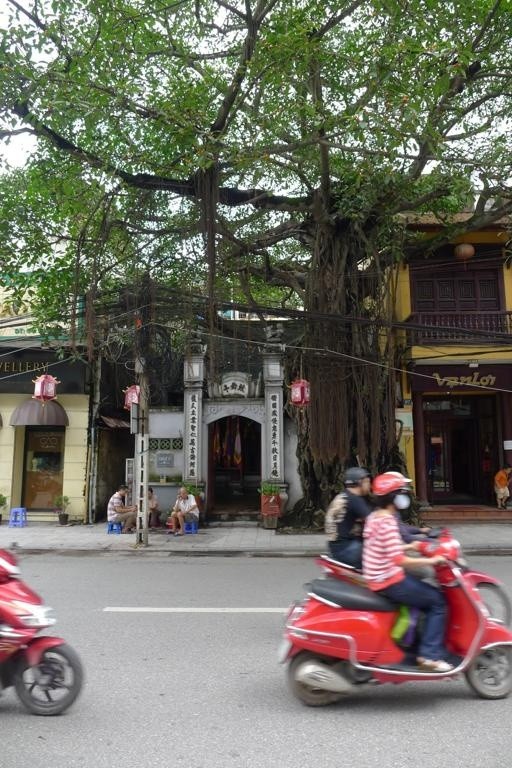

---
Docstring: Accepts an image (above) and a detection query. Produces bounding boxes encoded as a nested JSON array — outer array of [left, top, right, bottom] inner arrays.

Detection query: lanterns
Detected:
[[287, 379, 312, 413], [122, 384, 141, 410], [31, 373, 62, 414]]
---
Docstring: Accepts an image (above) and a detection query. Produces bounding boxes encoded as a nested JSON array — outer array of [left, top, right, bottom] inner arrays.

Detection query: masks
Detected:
[[395, 494, 411, 510]]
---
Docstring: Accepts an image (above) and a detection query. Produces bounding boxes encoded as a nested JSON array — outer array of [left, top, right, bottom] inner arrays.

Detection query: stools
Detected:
[[107, 521, 123, 535], [182, 520, 198, 534], [8, 506, 27, 528]]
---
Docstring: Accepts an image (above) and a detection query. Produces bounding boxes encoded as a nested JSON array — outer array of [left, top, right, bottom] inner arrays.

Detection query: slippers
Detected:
[[167, 531, 182, 536]]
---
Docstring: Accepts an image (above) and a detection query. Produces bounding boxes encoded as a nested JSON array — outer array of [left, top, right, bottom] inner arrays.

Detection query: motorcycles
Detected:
[[0, 543, 86, 716]]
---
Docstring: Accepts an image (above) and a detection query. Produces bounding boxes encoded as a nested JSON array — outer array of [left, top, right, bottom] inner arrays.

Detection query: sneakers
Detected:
[[122, 529, 133, 534], [498, 505, 507, 510], [417, 656, 453, 671]]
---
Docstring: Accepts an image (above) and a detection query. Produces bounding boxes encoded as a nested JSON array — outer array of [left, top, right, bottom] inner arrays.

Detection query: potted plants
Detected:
[[256, 482, 284, 516], [182, 484, 204, 510], [54, 495, 70, 525], [0, 493, 8, 525]]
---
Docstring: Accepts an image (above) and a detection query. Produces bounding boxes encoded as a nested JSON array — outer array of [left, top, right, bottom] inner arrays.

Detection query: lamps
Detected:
[[454, 242, 475, 259], [466, 359, 480, 368]]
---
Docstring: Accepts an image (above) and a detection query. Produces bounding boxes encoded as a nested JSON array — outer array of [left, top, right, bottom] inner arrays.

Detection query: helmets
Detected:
[[372, 472, 413, 497], [343, 467, 372, 483]]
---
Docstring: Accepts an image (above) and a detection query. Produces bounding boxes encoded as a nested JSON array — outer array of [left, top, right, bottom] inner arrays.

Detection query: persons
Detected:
[[324, 466, 371, 569], [493, 465, 511, 509], [362, 469, 454, 672], [107, 484, 137, 535], [146, 487, 162, 531], [167, 487, 200, 537], [381, 472, 443, 578]]
